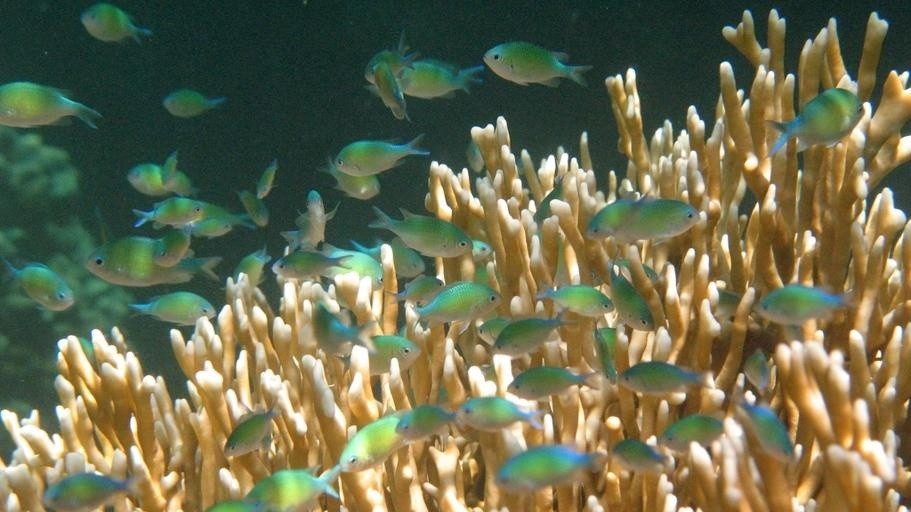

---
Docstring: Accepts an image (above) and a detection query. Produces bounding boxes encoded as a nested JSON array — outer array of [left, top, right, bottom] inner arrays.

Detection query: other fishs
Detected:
[[2, 2, 864, 512]]
